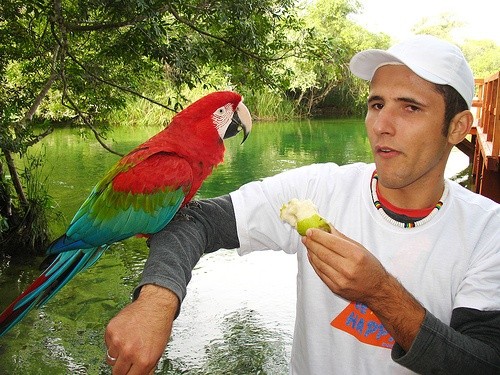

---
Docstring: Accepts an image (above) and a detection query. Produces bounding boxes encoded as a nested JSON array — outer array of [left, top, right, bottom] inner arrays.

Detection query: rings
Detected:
[[106, 349, 118, 362]]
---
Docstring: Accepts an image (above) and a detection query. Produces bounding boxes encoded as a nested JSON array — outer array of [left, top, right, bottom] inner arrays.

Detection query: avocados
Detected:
[[280, 200, 332, 236]]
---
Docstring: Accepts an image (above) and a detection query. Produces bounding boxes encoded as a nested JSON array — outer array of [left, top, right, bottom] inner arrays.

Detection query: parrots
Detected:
[[1, 90, 251, 336]]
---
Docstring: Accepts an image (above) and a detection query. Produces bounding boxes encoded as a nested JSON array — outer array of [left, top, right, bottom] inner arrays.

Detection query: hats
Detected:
[[345, 35, 476, 114]]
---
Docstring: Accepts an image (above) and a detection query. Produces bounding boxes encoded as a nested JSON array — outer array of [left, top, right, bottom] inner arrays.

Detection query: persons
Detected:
[[102, 33, 499, 374]]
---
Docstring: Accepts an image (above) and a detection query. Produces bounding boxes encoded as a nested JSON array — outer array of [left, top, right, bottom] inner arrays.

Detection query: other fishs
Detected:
[[72, 305, 89, 322], [77, 297, 115, 305], [98, 255, 123, 260], [73, 277, 107, 290], [45, 296, 75, 311], [101, 302, 121, 312], [95, 263, 122, 270]]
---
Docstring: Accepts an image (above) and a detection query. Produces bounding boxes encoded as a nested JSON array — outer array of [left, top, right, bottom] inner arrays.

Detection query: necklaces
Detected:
[[372, 170, 450, 228]]
